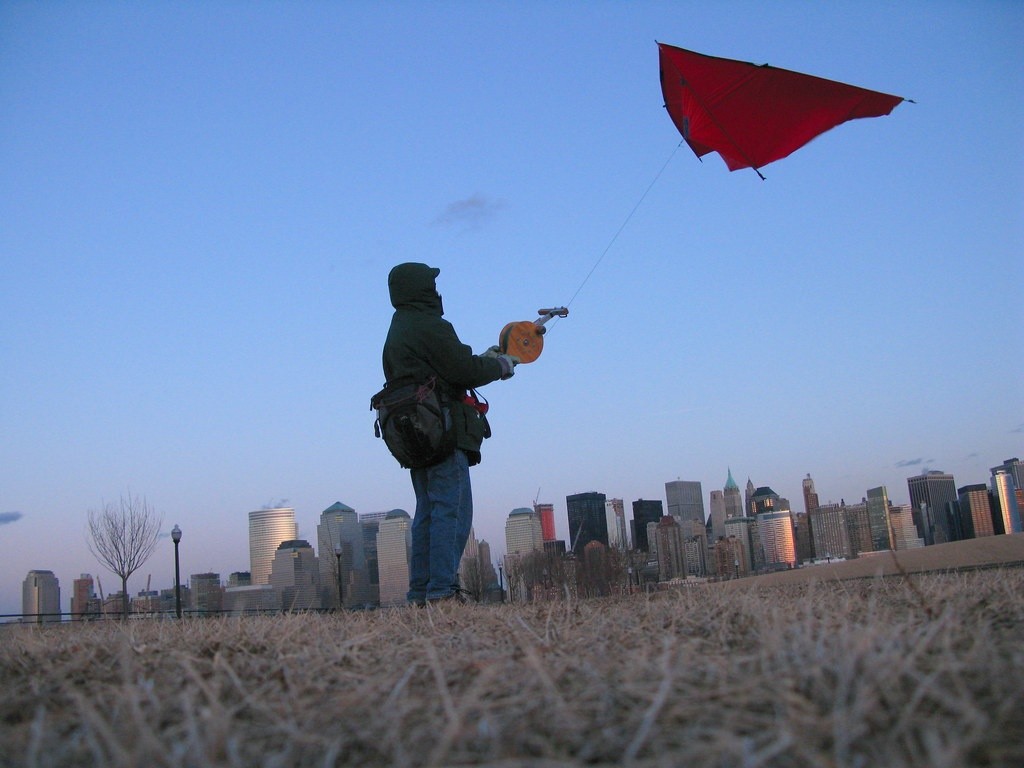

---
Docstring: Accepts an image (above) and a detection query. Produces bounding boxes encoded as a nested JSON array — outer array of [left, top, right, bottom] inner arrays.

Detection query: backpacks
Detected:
[[370, 376, 446, 469]]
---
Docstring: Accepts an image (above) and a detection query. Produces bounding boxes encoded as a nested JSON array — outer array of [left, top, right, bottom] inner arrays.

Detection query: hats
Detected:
[[431, 268, 440, 279]]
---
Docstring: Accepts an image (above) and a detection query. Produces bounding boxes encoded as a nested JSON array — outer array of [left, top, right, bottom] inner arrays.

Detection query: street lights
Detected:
[[334, 542, 343, 609], [171, 523, 182, 620], [735, 560, 739, 579], [627, 566, 632, 594], [497, 559, 504, 603]]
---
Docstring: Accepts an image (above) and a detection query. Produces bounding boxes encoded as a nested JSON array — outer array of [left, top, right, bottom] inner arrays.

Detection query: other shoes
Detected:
[[410, 587, 471, 608]]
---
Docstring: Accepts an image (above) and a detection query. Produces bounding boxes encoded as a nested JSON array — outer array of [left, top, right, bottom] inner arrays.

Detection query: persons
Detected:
[[383, 262, 521, 609]]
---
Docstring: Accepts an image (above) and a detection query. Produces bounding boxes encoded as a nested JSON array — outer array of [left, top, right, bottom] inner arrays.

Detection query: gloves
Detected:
[[479, 344, 501, 359], [496, 354, 520, 380]]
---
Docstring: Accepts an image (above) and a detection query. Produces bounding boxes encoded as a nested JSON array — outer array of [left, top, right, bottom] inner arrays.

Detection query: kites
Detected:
[[659, 42, 904, 172]]
